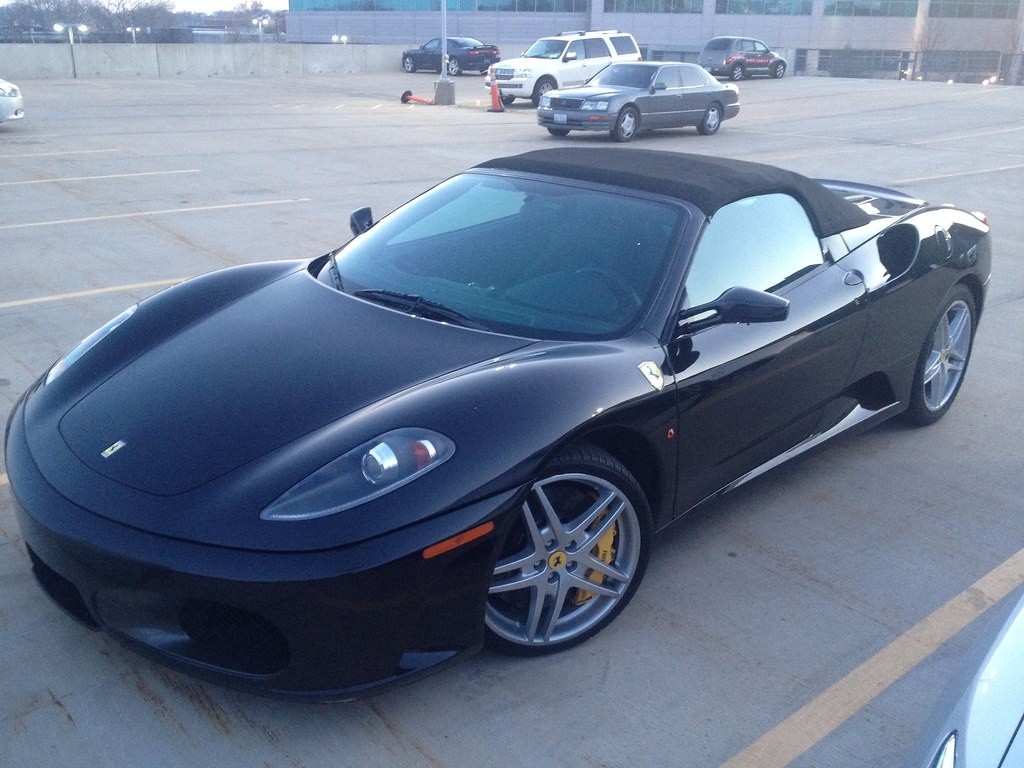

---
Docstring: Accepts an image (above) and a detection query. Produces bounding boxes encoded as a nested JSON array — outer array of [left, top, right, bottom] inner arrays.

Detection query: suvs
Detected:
[[485, 29, 644, 108]]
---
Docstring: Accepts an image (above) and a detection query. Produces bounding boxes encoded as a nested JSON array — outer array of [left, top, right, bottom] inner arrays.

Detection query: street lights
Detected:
[[127, 26, 140, 44], [253, 19, 269, 43], [54, 22, 89, 78]]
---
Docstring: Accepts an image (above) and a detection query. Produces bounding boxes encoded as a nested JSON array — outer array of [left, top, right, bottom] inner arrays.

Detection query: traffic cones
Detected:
[[486, 64, 506, 113], [400, 83, 436, 105]]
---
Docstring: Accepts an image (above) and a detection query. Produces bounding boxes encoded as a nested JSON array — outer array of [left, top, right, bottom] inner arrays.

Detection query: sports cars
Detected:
[[1, 136, 994, 709]]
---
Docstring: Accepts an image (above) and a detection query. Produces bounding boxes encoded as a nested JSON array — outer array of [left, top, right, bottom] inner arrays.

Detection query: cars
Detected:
[[698, 36, 788, 80], [0, 78, 25, 124], [537, 60, 742, 143], [401, 36, 501, 77]]
